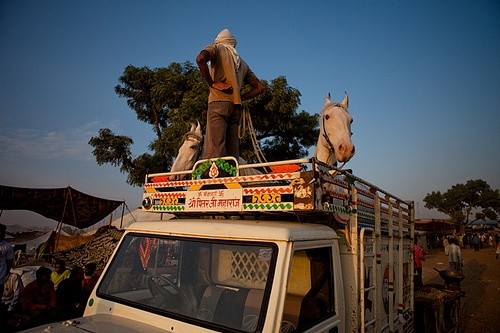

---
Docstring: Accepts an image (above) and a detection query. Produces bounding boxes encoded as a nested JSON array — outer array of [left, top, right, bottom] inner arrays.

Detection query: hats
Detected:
[[85, 263, 97, 272], [239, 142, 247, 150]]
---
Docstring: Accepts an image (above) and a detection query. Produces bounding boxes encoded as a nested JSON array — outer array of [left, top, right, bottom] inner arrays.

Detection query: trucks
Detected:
[[17, 155, 422, 333]]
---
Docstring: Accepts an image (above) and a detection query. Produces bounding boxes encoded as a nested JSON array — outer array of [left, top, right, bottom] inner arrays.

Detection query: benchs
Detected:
[[194, 283, 324, 333]]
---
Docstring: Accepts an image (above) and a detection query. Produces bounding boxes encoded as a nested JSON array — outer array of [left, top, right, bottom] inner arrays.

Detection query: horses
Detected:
[[168, 119, 278, 185], [313, 90, 356, 177]]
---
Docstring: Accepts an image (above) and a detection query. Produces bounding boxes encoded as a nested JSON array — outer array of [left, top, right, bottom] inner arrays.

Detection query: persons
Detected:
[[413, 237, 425, 286], [1, 269, 24, 319], [413, 231, 500, 250], [0, 223, 14, 301], [50, 259, 72, 293], [19, 266, 56, 326], [56, 266, 86, 321], [197, 28, 265, 168], [81, 259, 103, 300], [443, 235, 462, 276]]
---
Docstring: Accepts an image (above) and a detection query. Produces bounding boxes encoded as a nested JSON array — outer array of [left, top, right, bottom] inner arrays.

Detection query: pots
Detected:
[[439, 270, 464, 283]]
